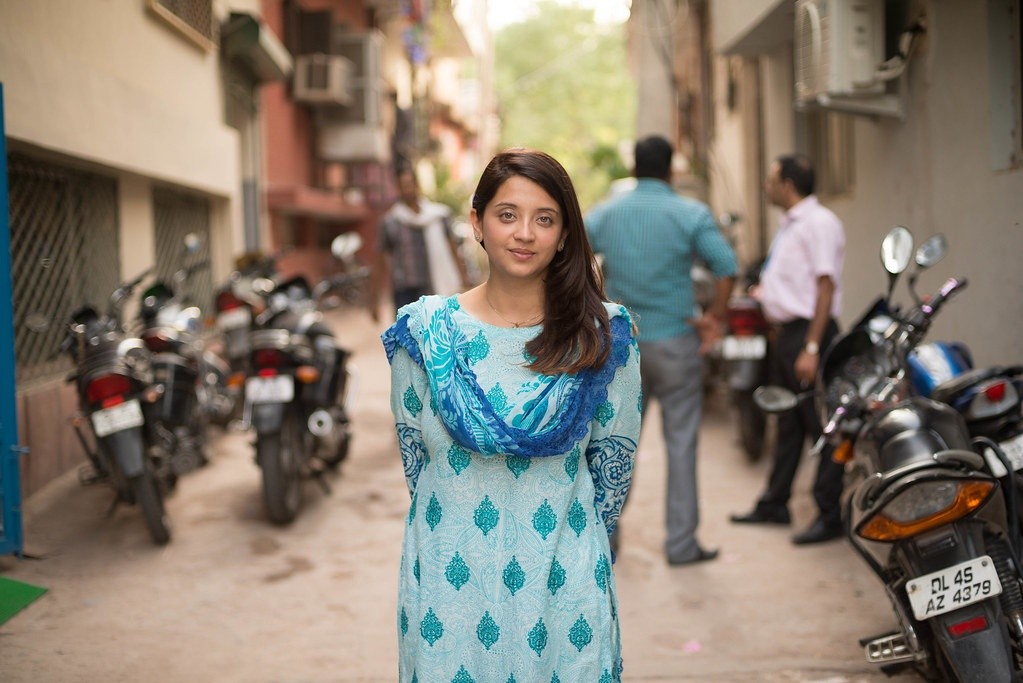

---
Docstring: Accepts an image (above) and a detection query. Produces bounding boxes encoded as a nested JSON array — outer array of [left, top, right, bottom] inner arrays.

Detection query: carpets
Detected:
[[0, 577, 47, 625]]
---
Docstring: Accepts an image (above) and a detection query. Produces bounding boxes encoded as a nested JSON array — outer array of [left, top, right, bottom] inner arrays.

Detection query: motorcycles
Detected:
[[689, 212, 768, 463], [754, 226, 1023, 683], [22, 233, 368, 547]]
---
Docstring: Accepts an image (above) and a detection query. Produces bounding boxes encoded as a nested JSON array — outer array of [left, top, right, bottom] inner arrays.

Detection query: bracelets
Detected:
[[802, 341, 820, 355]]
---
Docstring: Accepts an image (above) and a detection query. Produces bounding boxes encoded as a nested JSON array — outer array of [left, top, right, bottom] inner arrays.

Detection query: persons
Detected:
[[732, 153, 845, 543], [585, 134, 741, 564], [381, 148, 641, 682], [381, 169, 460, 311]]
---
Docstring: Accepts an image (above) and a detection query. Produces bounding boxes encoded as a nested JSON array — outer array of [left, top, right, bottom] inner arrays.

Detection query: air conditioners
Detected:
[[793, 0, 887, 111], [293, 55, 356, 107]]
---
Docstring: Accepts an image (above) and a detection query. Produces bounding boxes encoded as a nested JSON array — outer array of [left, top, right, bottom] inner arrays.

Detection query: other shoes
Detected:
[[731, 504, 790, 524], [793, 521, 837, 544], [699, 548, 719, 560]]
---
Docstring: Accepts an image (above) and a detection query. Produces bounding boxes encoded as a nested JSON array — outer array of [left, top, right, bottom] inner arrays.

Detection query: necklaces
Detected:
[[483, 281, 544, 327]]
